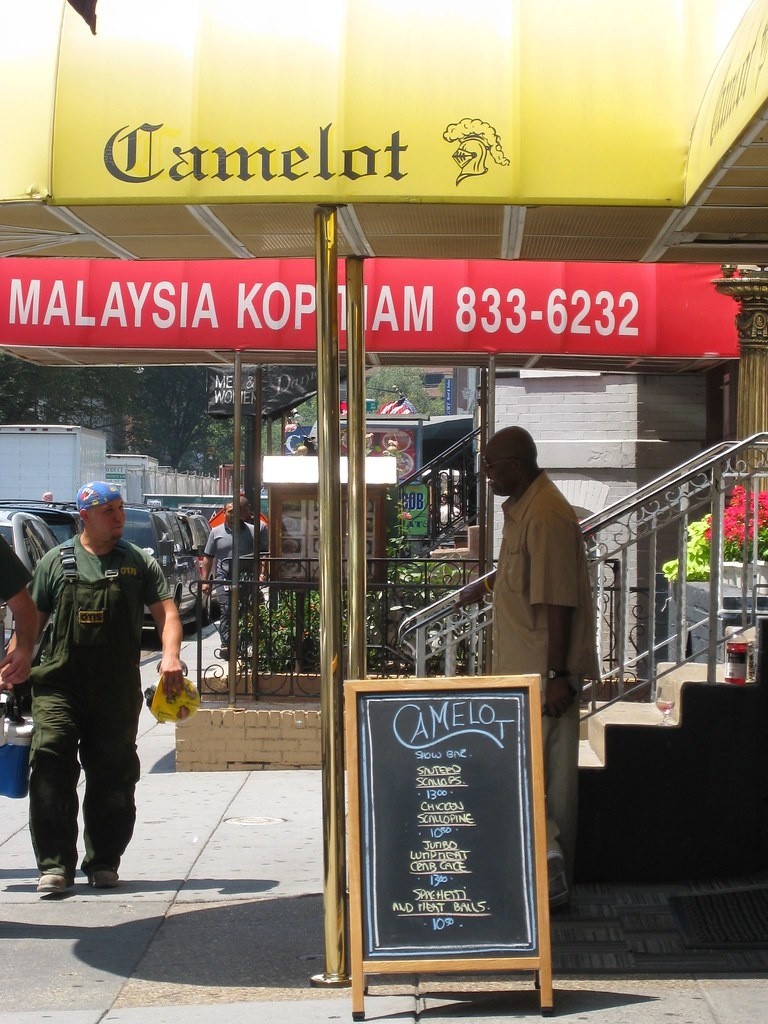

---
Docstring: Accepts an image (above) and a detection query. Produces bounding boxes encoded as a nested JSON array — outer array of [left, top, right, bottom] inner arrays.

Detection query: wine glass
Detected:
[[655, 685, 676, 727]]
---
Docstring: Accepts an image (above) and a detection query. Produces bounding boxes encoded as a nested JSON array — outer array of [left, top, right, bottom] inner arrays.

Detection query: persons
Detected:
[[42, 493, 53, 502], [0, 533, 40, 689], [483, 427, 599, 913], [202, 496, 269, 661], [0, 481, 184, 892]]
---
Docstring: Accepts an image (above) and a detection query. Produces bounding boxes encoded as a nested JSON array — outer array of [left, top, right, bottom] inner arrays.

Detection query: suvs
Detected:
[[0, 498, 216, 699]]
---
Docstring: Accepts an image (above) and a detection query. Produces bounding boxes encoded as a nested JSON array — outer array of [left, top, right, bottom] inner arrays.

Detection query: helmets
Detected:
[[151, 674, 201, 726]]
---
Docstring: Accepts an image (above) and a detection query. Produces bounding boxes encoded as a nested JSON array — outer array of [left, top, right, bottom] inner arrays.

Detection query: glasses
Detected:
[[482, 457, 505, 469]]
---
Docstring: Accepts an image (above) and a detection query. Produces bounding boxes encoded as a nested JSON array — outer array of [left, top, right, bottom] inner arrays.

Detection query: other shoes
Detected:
[[37, 873, 68, 892], [220, 646, 229, 661], [549, 871, 573, 911], [88, 870, 119, 887]]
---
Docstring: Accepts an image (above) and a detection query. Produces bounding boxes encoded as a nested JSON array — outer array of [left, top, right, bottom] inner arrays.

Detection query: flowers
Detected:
[[704, 484, 768, 565]]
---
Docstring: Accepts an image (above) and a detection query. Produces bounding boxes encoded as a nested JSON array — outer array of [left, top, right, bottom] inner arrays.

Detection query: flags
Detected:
[[375, 398, 417, 415]]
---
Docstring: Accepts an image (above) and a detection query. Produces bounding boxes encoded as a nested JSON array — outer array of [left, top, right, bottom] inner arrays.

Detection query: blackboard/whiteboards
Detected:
[[340, 674, 552, 976]]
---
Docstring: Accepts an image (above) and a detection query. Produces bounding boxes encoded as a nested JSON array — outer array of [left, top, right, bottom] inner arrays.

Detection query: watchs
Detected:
[[546, 669, 570, 679]]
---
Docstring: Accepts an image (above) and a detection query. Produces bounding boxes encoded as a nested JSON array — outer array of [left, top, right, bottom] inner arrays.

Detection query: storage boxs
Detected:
[[0, 717, 34, 798]]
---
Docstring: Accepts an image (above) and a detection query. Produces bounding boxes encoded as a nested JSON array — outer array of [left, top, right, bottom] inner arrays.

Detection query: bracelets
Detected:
[[260, 574, 264, 576]]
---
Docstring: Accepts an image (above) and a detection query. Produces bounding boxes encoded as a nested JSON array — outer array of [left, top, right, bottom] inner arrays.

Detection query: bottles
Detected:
[[725, 628, 748, 686]]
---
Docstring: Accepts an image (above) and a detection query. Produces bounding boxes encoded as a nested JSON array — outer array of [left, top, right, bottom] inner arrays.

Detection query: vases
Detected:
[[722, 562, 768, 595]]
